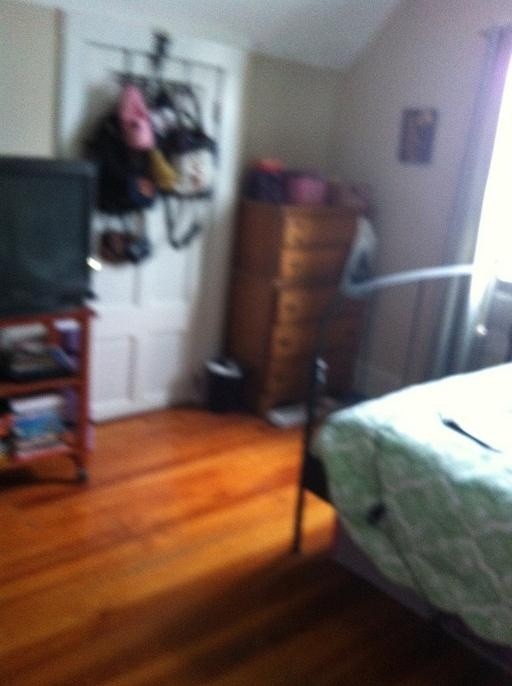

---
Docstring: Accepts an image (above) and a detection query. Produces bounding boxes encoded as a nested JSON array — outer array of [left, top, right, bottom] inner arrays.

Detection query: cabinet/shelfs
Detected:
[[0, 306, 86, 472], [226, 196, 356, 414]]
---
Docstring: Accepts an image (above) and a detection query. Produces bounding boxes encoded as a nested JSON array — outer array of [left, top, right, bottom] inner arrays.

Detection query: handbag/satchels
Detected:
[[165, 126, 219, 199]]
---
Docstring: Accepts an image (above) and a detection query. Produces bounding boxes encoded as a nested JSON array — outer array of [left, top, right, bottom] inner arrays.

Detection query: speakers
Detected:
[[1, 157, 96, 318]]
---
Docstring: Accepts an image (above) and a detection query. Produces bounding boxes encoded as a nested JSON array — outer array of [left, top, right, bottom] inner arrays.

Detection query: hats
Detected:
[[119, 88, 154, 149]]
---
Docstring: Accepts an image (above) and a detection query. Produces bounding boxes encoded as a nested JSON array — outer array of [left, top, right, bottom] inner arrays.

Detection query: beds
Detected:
[[311, 362, 512, 648]]
